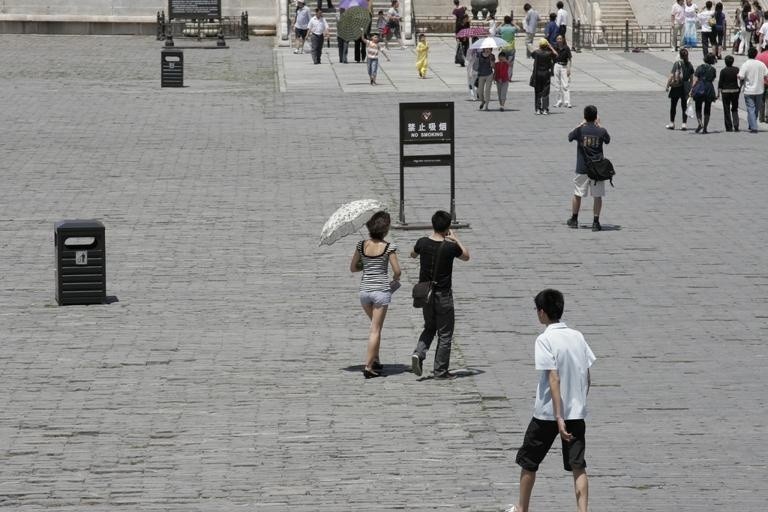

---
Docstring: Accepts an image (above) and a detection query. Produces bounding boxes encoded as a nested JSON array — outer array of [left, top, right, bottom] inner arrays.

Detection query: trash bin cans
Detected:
[[161, 50, 184, 87], [55, 220, 106, 305]]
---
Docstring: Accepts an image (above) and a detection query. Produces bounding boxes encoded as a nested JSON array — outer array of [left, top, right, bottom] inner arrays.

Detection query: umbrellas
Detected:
[[318, 199, 388, 247]]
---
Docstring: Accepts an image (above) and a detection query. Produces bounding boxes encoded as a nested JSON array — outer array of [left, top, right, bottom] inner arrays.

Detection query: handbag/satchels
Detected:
[[586, 158, 615, 181], [412, 280, 436, 308], [668, 71, 680, 87], [690, 81, 707, 99]]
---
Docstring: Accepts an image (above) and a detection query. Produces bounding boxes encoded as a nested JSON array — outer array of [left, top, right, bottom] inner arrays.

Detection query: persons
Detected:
[[666, 0, 768, 134], [566, 106, 610, 231], [410, 211, 470, 380], [350, 211, 401, 378], [293, 0, 573, 115], [504, 288, 597, 512]]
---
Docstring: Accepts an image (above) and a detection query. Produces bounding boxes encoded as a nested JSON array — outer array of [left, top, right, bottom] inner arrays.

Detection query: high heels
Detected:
[[364, 360, 384, 379]]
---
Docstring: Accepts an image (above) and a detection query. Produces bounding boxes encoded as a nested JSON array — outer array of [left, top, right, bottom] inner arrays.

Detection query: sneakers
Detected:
[[567, 219, 578, 227], [412, 351, 422, 376], [434, 371, 458, 380], [592, 222, 601, 231]]
